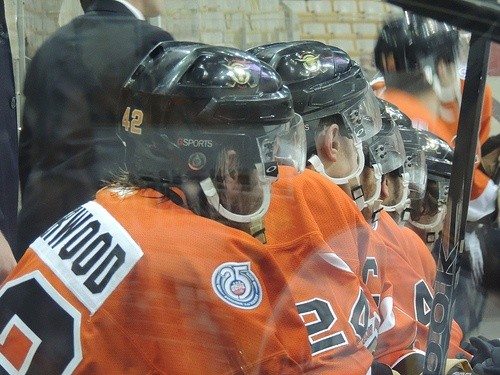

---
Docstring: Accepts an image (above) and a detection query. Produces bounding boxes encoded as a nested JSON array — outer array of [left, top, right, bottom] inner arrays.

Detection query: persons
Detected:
[[13, 1, 176, 261], [364, 16, 499, 374], [1, 0, 19, 289], [243, 40, 383, 374], [1, 40, 317, 375]]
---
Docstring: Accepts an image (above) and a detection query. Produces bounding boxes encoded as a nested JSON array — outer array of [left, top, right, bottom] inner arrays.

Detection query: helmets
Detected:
[[410, 125, 457, 201], [361, 97, 405, 176], [372, 97, 423, 172], [245, 40, 381, 167], [115, 39, 296, 179], [373, 18, 459, 93]]
